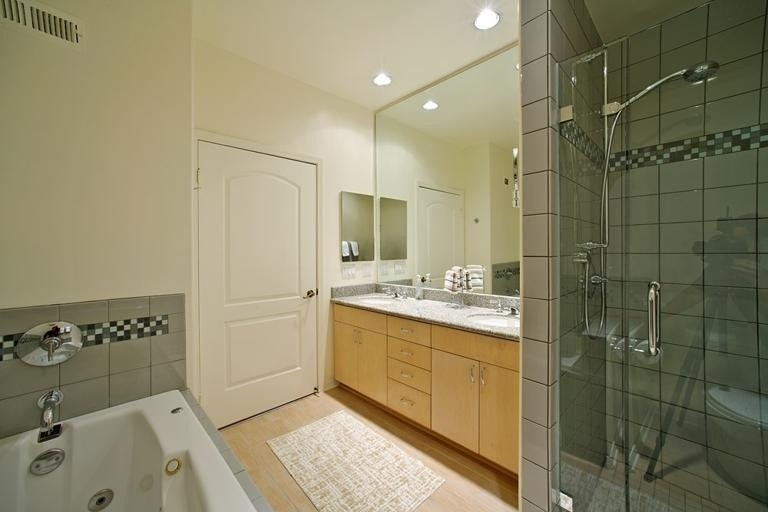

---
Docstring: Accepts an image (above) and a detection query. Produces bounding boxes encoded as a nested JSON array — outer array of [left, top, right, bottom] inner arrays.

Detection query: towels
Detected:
[[443, 264, 484, 292]]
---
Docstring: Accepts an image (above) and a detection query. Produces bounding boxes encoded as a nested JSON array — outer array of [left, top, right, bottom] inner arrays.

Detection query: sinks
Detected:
[[466, 313, 521, 329], [360, 297, 401, 304]]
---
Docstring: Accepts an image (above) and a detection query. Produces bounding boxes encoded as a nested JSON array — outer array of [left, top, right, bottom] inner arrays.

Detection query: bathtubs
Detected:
[[0, 388, 258, 512]]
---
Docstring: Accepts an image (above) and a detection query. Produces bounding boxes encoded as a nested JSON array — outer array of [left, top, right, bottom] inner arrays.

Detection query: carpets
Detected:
[[266, 410, 445, 512]]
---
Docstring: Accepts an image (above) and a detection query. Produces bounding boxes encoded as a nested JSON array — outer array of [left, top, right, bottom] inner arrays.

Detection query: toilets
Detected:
[[705, 385, 768, 498]]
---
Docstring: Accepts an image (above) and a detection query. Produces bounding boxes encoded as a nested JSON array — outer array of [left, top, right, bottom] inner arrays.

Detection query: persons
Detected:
[[631, 213, 766, 353]]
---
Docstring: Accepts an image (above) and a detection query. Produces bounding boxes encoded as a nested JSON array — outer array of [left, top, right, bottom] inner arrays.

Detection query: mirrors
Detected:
[[373, 41, 522, 300]]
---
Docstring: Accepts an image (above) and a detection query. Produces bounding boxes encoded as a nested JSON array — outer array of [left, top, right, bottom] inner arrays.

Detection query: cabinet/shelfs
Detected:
[[430, 323, 520, 482], [333, 301, 388, 413], [386, 314, 431, 435]]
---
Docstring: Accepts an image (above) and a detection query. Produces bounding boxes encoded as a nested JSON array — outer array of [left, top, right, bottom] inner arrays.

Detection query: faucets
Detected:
[[37, 389, 64, 437], [393, 291, 401, 298], [511, 306, 519, 316]]
